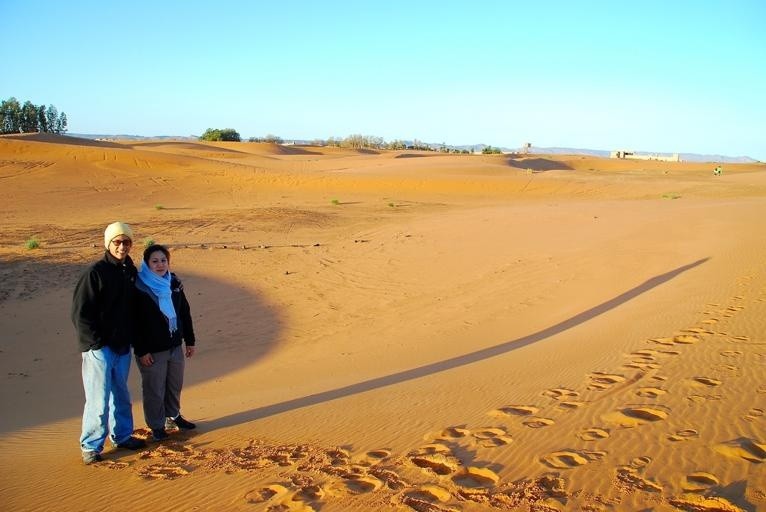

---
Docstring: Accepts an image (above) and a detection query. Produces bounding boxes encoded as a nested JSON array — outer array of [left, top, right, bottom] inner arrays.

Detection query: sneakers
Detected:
[[166, 415, 195, 430], [82, 452, 100, 465], [151, 428, 168, 441], [117, 437, 145, 448]]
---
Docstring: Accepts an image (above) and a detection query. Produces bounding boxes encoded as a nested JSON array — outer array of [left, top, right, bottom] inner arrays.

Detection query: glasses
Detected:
[[111, 240, 131, 246]]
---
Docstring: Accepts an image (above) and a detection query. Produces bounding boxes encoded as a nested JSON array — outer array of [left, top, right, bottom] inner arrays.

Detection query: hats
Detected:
[[104, 222, 132, 251]]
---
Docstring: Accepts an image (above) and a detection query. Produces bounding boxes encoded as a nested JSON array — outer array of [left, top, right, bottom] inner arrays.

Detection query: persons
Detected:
[[70, 222, 184, 465], [131, 244, 195, 440]]
